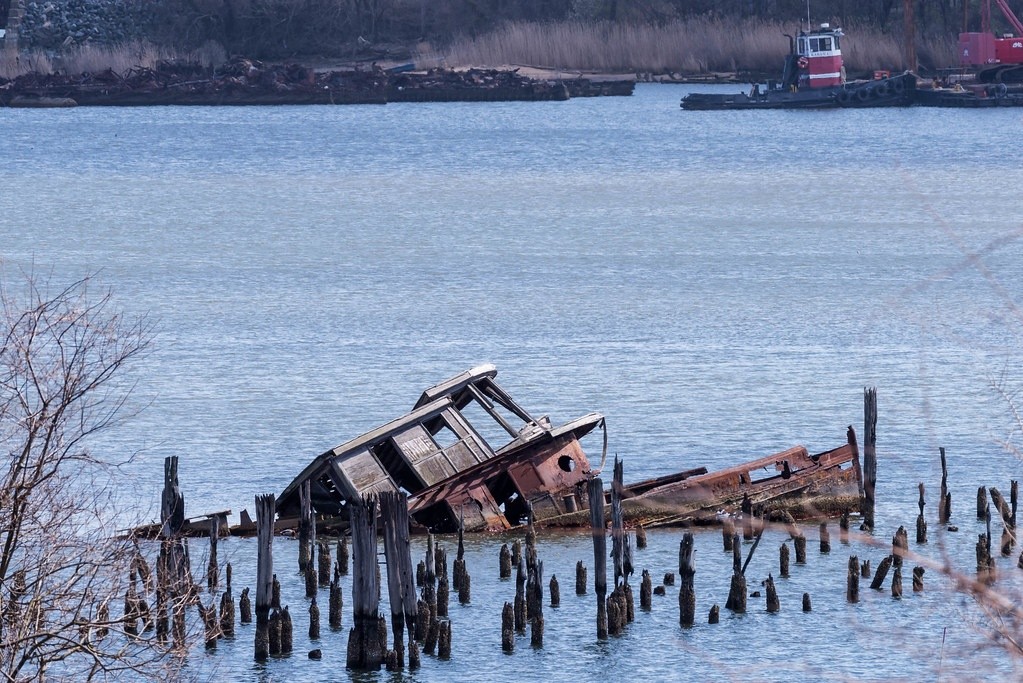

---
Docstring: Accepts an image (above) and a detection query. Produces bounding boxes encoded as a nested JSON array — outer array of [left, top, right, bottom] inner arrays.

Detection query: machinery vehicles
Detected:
[[955, 0, 1023, 65]]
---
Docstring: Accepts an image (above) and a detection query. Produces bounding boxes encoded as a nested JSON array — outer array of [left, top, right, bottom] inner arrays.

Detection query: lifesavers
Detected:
[[797, 56, 809, 69]]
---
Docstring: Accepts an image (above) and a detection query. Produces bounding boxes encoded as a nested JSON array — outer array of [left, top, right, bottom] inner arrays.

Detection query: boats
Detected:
[[677, 1, 920, 111], [913, 85, 1023, 108]]
